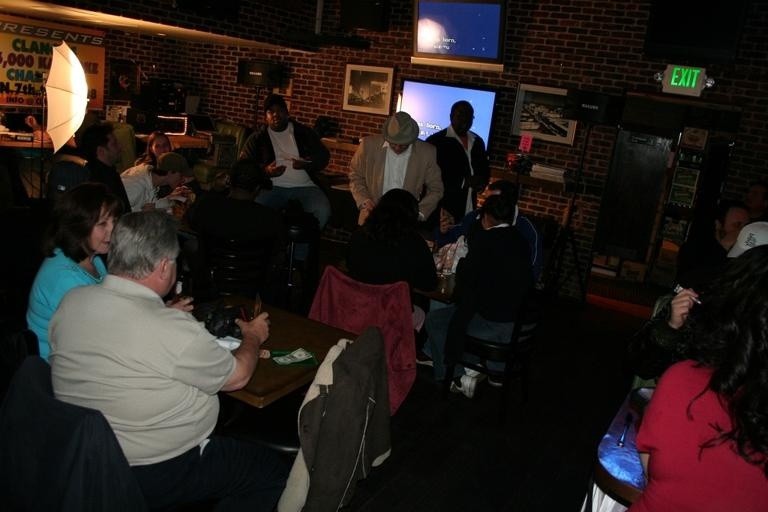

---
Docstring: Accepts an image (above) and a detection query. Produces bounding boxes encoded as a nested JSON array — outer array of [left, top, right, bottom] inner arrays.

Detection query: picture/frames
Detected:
[[108, 57, 142, 97], [342, 61, 396, 117], [510, 82, 578, 147]]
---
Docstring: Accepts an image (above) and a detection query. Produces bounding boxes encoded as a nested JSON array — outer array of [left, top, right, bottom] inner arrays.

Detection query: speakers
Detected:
[[343, 0, 388, 33], [561, 88, 622, 126], [236, 60, 284, 89]]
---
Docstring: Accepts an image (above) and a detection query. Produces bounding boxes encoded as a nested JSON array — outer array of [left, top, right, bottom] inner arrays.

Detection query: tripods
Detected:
[[536, 127, 592, 310]]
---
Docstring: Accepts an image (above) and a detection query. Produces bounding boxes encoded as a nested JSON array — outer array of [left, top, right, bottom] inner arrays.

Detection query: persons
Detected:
[[22, 90, 546, 511], [745, 181, 768, 223], [625, 219, 768, 379], [625, 245, 766, 512], [673, 200, 749, 294]]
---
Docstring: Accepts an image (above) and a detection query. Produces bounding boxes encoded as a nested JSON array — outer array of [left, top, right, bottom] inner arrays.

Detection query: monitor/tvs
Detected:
[[644, 0, 749, 66], [412, 0, 507, 64], [395, 76, 499, 155]]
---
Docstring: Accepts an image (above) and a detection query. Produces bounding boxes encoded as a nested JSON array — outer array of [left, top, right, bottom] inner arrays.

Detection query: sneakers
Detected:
[[450, 363, 503, 398], [415, 349, 434, 367]]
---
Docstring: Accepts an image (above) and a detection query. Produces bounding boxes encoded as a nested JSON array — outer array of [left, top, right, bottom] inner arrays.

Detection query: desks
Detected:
[[591, 359, 668, 511]]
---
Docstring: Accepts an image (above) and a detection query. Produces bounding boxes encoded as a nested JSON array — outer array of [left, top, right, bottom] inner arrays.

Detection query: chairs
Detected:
[[1, 120, 248, 173]]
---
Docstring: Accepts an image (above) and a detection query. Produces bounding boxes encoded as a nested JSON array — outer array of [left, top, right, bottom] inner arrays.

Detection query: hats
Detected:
[[727, 221, 768, 258], [158, 151, 192, 176], [383, 112, 419, 144], [263, 94, 285, 111]]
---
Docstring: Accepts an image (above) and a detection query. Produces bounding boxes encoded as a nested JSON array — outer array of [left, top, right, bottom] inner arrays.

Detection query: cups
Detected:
[[423, 239, 456, 279], [240, 297, 263, 323]]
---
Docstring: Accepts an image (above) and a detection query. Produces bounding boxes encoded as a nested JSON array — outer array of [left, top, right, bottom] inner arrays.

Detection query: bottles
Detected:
[[173, 272, 187, 305]]
